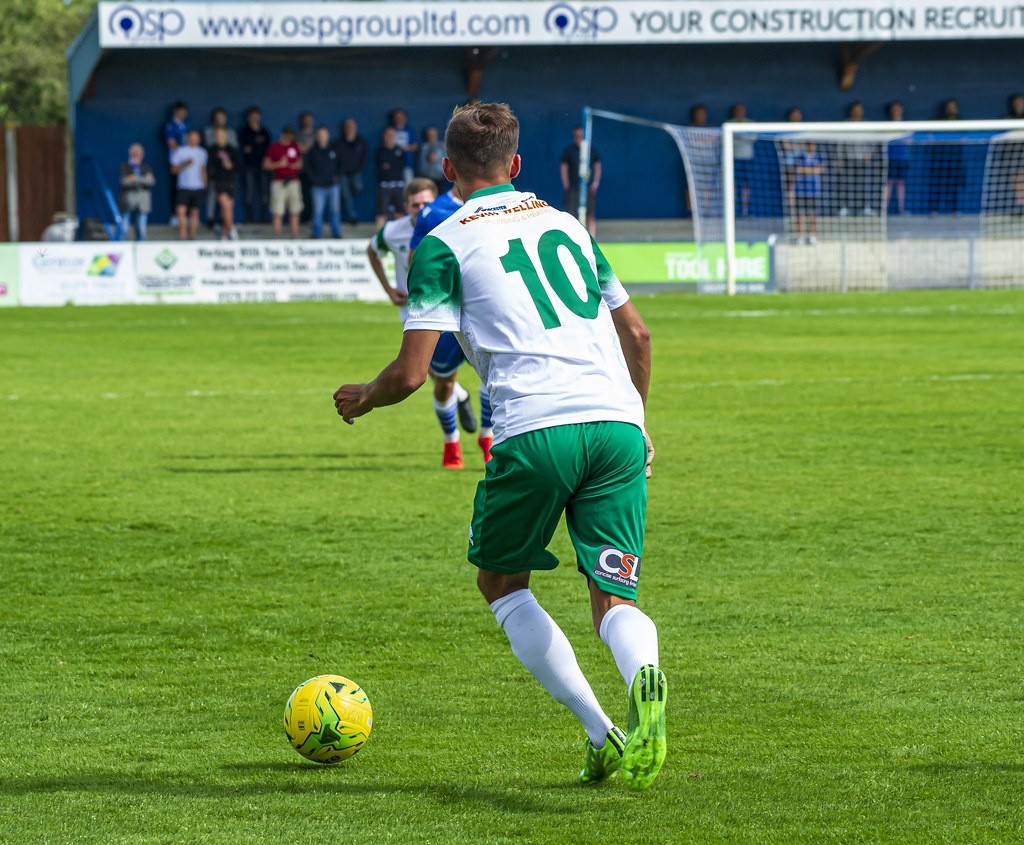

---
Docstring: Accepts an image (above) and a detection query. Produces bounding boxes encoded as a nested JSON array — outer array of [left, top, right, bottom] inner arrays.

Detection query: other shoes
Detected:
[[808, 236, 817, 245], [478, 435, 493, 462], [458, 390, 476, 433], [796, 237, 804, 246], [442, 440, 465, 470]]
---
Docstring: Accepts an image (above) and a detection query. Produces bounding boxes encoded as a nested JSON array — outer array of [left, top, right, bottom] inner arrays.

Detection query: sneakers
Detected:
[[580, 726, 628, 783], [622, 663, 668, 790]]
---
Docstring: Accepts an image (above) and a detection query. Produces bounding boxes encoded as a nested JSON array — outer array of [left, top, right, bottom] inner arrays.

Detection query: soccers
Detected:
[[283, 674, 373, 764]]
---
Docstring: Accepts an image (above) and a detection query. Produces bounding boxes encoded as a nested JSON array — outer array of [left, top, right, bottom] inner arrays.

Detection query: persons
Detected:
[[680, 104, 759, 219], [998, 93, 1024, 216], [779, 107, 825, 246], [164, 101, 447, 239], [367, 178, 494, 470], [928, 100, 967, 216], [115, 142, 155, 241], [560, 123, 601, 238], [832, 101, 878, 218], [332, 99, 667, 791], [878, 100, 912, 219]]
